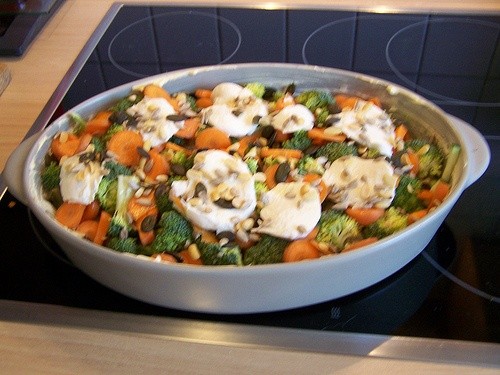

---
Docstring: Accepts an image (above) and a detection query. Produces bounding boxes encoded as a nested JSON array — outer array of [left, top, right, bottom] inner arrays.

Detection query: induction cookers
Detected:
[[0, 0, 500, 369]]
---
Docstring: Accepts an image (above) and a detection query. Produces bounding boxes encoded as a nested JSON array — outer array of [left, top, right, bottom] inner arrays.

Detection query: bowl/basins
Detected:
[[3, 62, 490, 312]]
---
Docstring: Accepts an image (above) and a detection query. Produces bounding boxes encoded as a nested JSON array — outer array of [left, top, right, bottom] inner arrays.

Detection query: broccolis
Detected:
[[41, 83, 461, 266]]
